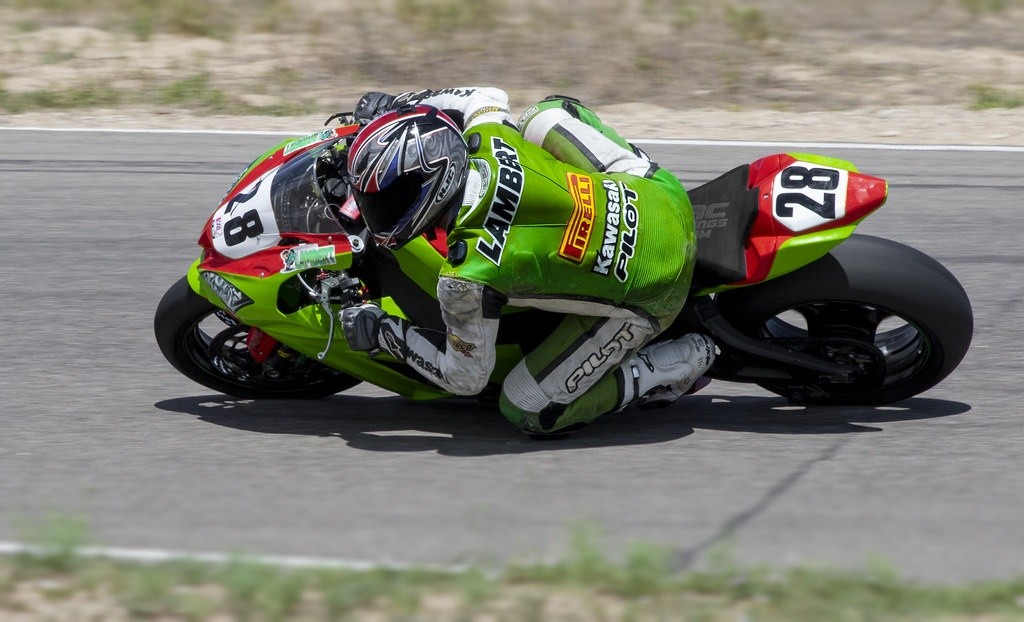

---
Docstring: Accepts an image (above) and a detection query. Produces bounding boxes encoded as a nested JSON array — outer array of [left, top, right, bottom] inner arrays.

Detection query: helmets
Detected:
[[348, 104, 468, 251]]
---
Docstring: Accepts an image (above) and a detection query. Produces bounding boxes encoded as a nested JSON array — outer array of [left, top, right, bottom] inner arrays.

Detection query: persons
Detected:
[[340, 85, 717, 438]]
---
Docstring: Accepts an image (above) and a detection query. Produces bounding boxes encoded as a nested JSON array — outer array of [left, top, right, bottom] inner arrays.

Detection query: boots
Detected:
[[614, 334, 715, 414]]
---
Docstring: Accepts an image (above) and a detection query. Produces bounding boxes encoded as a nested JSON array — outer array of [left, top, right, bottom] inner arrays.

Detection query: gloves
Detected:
[[338, 304, 387, 352], [355, 92, 397, 124]]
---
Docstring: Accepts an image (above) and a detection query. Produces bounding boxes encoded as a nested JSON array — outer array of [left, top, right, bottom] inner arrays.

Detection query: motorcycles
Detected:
[[153, 111, 974, 400]]
